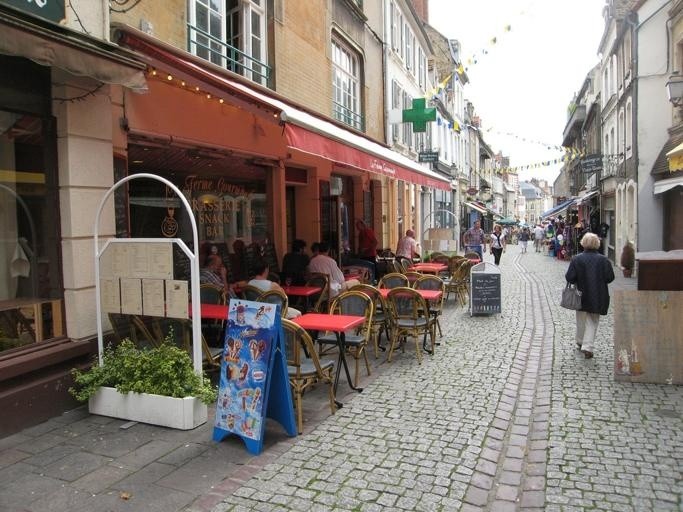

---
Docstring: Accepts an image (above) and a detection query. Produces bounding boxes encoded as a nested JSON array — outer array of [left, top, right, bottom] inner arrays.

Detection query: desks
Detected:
[[290, 313, 365, 408]]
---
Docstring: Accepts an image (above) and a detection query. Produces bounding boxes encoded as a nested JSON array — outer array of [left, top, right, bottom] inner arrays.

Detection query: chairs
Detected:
[[108, 254, 474, 365], [314, 291, 373, 389], [280, 317, 336, 434], [160, 313, 222, 392]]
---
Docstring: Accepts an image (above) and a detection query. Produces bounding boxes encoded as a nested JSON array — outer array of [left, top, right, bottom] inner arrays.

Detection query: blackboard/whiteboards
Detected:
[[468, 271, 503, 314]]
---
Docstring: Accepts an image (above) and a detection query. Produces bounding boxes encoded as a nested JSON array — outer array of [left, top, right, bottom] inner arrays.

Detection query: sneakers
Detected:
[[585, 351, 593, 359]]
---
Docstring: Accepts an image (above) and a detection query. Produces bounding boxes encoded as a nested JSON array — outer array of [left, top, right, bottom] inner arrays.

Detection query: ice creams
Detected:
[[228, 337, 242, 358], [249, 339, 266, 360]]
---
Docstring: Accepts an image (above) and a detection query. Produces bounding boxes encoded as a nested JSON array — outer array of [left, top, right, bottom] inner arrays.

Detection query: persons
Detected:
[[200, 218, 417, 320], [565, 232, 615, 359], [462, 215, 565, 265]]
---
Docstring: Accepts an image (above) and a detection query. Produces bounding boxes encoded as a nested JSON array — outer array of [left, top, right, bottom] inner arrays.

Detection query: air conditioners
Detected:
[[330, 177, 342, 196]]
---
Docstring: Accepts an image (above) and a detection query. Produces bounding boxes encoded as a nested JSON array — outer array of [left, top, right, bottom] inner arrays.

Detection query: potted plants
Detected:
[[65, 334, 214, 430]]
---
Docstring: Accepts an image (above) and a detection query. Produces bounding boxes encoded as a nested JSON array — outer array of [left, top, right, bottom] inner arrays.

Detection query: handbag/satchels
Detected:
[[561, 288, 582, 311]]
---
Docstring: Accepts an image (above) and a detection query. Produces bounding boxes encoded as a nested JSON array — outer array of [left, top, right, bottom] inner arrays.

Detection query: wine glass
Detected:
[[168, 207, 174, 219]]
